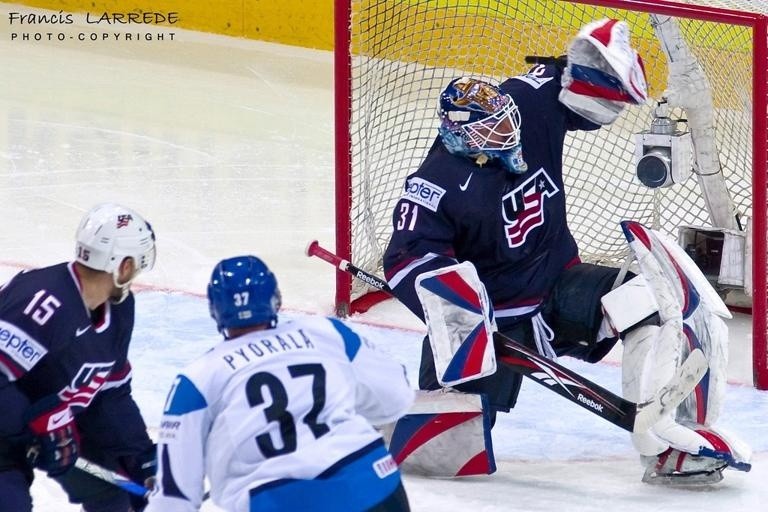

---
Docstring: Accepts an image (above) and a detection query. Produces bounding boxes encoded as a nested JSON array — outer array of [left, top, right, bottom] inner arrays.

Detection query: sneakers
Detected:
[[640, 447, 729, 474]]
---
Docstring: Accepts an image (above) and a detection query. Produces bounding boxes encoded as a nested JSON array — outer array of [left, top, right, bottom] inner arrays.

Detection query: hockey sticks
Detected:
[[305, 239, 708, 434]]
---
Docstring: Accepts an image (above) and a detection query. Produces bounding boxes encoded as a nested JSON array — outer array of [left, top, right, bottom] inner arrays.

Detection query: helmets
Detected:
[[436, 74, 528, 175], [206, 255, 283, 334], [75, 203, 156, 290]]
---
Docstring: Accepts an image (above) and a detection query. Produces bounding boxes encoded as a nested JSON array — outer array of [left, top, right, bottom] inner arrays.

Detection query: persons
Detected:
[[147, 255, 413, 512], [386, 14, 739, 491], [0, 198, 172, 511]]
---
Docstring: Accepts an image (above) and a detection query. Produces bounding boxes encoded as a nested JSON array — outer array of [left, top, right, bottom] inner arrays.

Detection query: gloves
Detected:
[[27, 400, 82, 478], [117, 443, 156, 511]]
